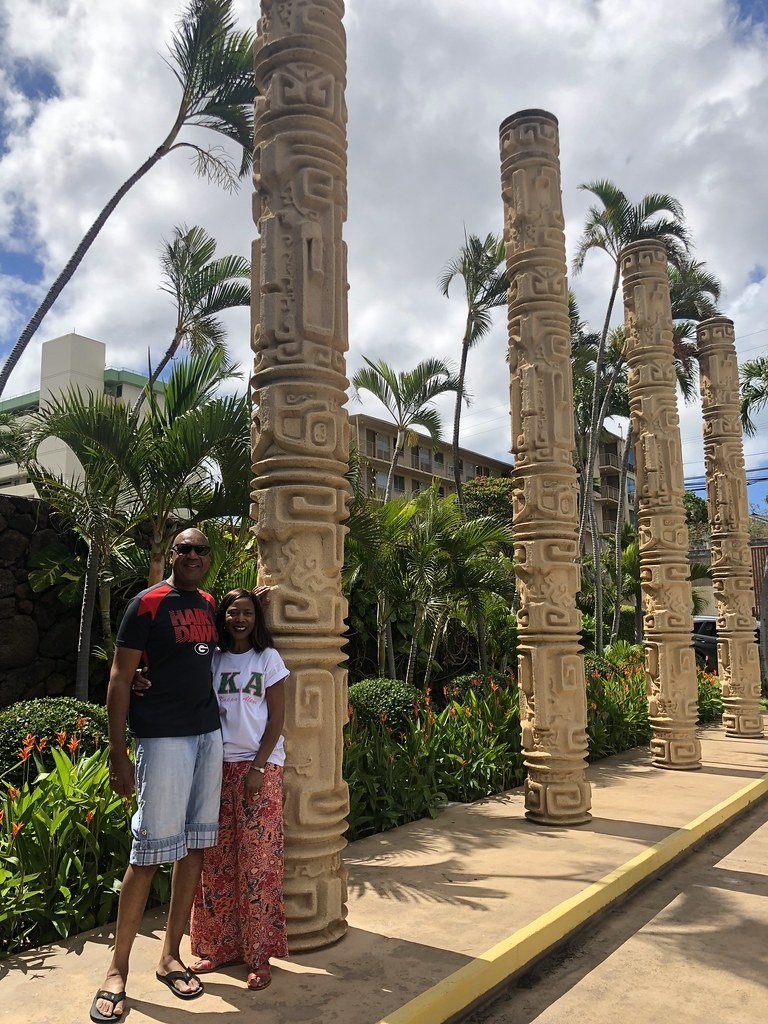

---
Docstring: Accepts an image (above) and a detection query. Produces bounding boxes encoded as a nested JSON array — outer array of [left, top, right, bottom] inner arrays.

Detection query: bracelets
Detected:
[[250, 764, 265, 773]]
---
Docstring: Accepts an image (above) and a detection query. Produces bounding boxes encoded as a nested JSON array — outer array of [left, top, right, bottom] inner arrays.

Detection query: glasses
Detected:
[[172, 543, 212, 556]]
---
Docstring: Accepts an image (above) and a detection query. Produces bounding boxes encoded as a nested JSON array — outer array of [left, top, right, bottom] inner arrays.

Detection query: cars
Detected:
[[691, 614, 761, 673]]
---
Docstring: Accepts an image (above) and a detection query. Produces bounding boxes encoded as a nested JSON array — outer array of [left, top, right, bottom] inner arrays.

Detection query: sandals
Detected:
[[191, 956, 247, 973], [248, 961, 272, 990]]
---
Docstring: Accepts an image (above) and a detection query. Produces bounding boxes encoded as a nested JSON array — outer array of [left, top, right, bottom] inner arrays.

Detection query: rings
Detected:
[[132, 684, 135, 690], [109, 774, 118, 780]]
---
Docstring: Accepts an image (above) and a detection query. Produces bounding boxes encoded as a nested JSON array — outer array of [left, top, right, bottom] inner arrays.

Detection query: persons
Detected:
[[131, 589, 290, 990], [90, 528, 224, 1024]]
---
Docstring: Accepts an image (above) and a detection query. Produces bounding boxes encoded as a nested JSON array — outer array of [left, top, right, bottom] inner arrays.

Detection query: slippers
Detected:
[[156, 965, 204, 999], [90, 987, 126, 1023]]
